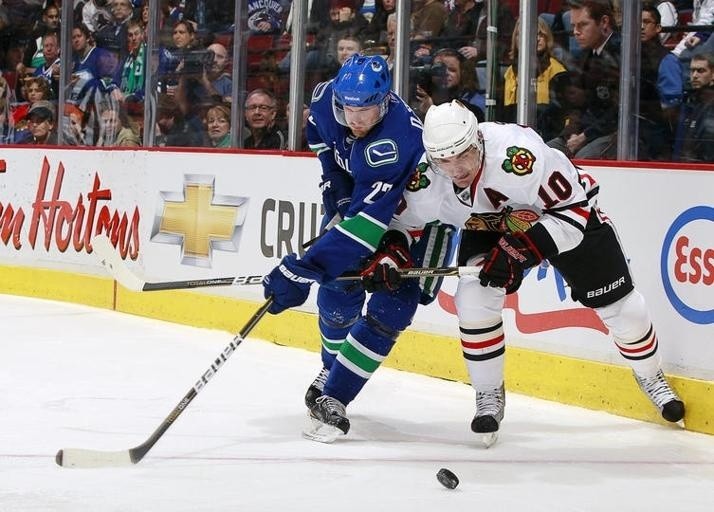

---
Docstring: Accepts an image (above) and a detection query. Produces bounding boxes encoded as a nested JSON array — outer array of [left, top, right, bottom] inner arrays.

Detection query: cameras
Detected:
[[409, 55, 448, 103], [181, 49, 216, 75]]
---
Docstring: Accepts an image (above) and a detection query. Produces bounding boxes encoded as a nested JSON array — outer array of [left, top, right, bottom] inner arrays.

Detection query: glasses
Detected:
[[245, 104, 273, 111]]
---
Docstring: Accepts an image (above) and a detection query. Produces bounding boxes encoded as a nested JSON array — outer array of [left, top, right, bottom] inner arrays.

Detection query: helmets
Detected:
[[423, 99, 484, 182], [333, 53, 391, 128]]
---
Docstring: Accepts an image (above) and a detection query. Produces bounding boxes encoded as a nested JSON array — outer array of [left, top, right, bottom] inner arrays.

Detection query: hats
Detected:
[[26, 106, 53, 123]]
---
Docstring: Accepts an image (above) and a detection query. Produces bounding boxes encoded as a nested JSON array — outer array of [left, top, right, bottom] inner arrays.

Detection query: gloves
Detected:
[[262, 253, 319, 315], [362, 229, 412, 292], [479, 230, 541, 293], [321, 178, 356, 218]]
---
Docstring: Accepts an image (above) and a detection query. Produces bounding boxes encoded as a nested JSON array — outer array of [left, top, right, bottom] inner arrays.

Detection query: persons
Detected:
[[364, 100, 686, 449], [0, 0, 714, 164], [263, 53, 456, 444]]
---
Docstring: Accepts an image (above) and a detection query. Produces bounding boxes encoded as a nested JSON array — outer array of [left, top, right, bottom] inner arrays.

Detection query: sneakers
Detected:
[[470, 384, 505, 433], [310, 394, 350, 435], [632, 367, 686, 422], [305, 367, 330, 408]]
